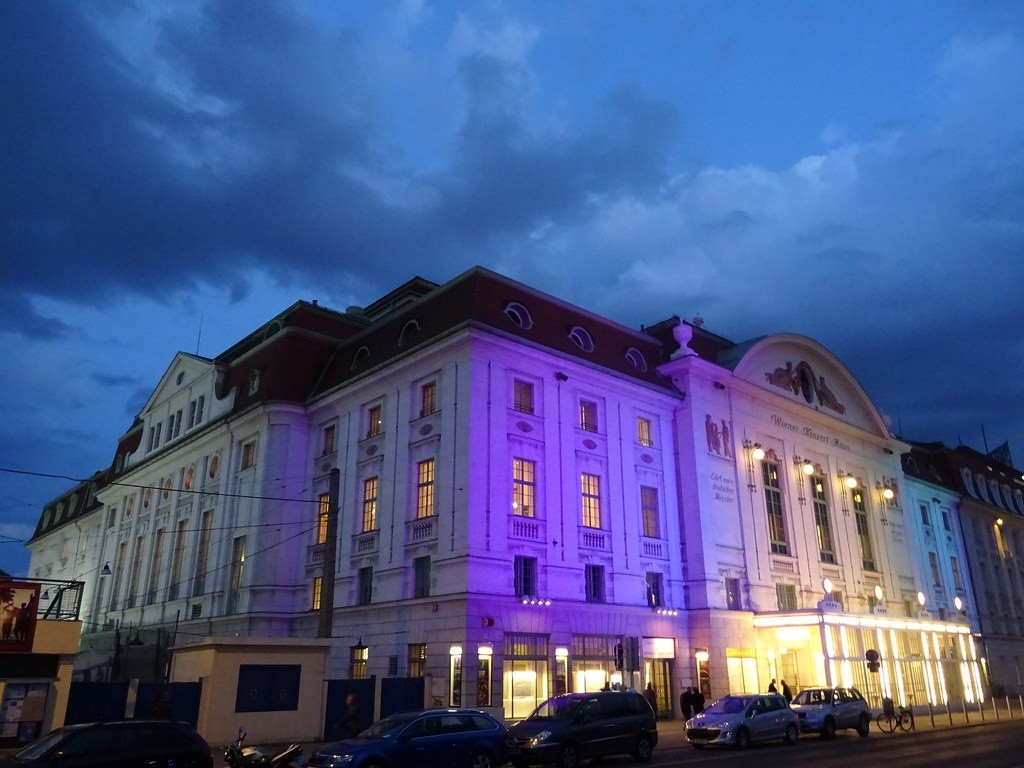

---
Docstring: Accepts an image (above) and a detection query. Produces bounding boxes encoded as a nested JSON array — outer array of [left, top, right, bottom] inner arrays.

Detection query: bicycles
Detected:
[[877, 705, 913, 733]]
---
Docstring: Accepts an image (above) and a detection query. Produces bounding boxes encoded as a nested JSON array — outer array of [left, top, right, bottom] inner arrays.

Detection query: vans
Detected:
[[507, 692, 659, 768]]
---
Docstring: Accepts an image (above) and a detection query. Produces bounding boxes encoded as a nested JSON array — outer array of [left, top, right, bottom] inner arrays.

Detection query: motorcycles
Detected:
[[217, 726, 308, 768]]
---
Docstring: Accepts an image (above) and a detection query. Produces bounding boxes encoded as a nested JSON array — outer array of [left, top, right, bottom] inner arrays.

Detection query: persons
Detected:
[[813, 375, 847, 414], [705, 415, 732, 457], [763, 360, 799, 392], [643, 682, 658, 723], [680, 686, 706, 732], [781, 678, 792, 705], [600, 681, 612, 691], [768, 678, 778, 693], [2, 603, 30, 641]]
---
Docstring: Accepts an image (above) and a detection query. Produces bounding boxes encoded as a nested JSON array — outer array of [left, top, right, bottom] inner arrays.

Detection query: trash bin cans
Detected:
[[881, 697, 894, 719]]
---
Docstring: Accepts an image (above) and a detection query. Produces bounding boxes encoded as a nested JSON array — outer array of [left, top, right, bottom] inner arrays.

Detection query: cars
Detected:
[[684, 692, 801, 752], [308, 707, 508, 768], [789, 685, 872, 741]]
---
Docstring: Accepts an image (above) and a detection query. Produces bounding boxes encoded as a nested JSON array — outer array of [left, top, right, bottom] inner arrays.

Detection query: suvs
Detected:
[[0, 718, 214, 768]]
